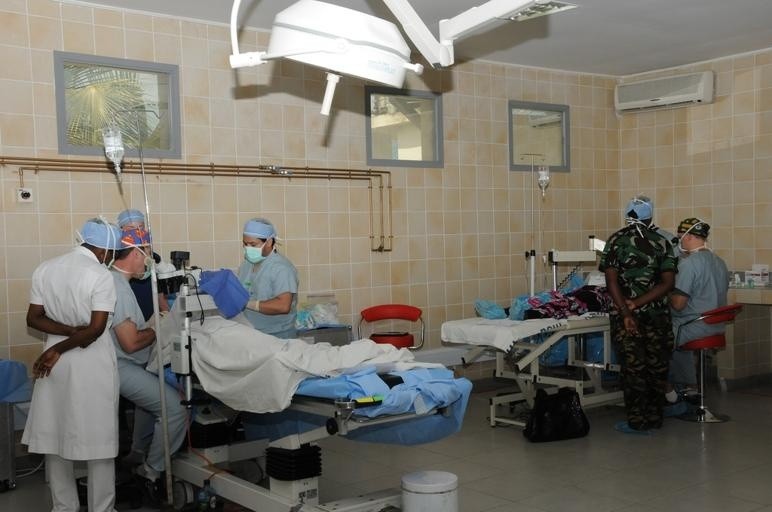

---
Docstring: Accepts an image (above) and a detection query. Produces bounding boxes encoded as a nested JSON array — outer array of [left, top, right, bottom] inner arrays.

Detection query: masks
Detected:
[[141, 257, 152, 281], [677, 239, 683, 252], [242, 246, 267, 265]]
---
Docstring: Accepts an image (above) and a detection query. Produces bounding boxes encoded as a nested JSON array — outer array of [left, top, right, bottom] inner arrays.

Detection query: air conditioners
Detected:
[[614, 69, 716, 115]]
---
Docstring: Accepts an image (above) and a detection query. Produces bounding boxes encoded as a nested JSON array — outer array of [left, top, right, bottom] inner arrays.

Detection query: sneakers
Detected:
[[613, 399, 686, 435], [134, 466, 164, 500]]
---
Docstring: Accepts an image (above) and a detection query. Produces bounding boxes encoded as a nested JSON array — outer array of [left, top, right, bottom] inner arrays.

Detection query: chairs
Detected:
[[356, 303, 426, 354], [670, 298, 747, 426]]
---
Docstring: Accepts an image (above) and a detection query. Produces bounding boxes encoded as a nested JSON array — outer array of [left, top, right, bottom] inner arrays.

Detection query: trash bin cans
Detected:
[[400, 470, 459, 512]]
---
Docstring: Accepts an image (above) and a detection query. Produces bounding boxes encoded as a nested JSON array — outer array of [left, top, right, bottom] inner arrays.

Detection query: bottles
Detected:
[[197, 478, 217, 511]]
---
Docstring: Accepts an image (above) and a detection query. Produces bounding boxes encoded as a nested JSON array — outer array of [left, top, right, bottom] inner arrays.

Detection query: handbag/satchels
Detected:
[[523, 387, 589, 442]]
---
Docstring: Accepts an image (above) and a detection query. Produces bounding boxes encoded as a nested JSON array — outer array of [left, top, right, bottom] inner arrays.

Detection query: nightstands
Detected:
[[298, 325, 352, 348]]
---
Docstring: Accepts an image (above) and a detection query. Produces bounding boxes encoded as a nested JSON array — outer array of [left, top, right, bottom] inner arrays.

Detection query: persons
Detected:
[[599, 194, 733, 437], [233, 216, 301, 340], [79, 209, 191, 501], [25, 218, 120, 511], [475, 281, 611, 320]]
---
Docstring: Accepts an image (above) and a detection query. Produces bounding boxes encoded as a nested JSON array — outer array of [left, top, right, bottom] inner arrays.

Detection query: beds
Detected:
[[438, 269, 628, 434], [138, 311, 474, 512]]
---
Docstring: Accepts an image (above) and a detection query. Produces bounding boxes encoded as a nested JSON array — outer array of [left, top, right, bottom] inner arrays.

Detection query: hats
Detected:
[[678, 218, 710, 238], [79, 209, 152, 251], [243, 218, 277, 240]]
